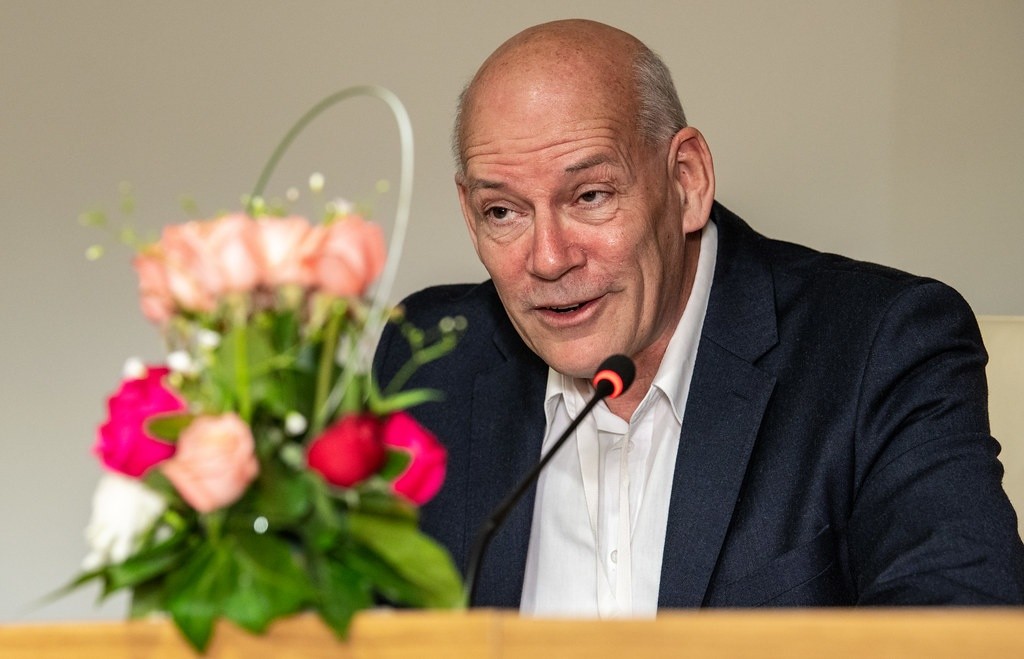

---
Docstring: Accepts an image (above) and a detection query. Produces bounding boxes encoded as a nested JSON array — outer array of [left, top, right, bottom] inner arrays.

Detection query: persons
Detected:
[[370, 18, 1024, 611]]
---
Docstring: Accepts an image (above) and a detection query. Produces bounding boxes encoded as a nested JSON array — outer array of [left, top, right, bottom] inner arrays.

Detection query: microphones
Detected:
[[462, 354, 636, 609]]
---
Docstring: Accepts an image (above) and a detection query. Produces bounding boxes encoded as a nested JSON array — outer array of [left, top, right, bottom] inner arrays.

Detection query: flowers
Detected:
[[48, 169, 474, 657]]
